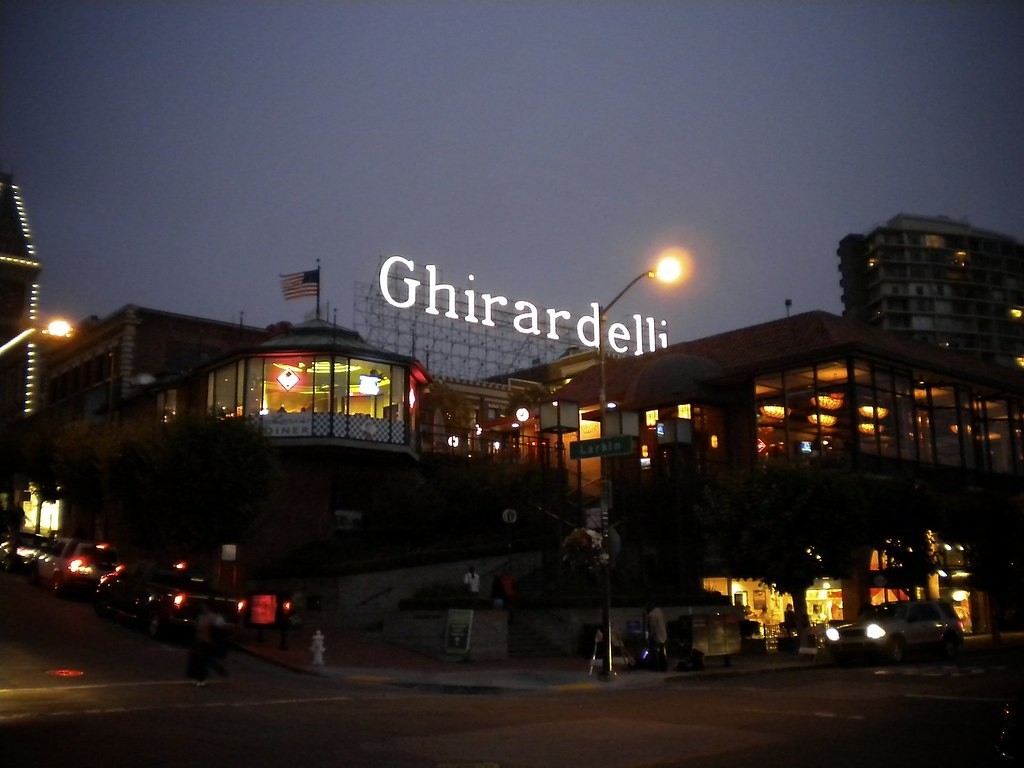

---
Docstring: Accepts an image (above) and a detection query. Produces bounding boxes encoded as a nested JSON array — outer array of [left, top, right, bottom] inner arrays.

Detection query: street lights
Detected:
[[598, 257, 686, 681]]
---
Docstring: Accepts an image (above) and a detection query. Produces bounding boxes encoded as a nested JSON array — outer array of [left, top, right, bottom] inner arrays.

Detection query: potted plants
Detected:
[[777, 611, 802, 653]]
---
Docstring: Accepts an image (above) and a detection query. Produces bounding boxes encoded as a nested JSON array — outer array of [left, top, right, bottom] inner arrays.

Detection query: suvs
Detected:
[[37, 539, 124, 593], [828, 598, 965, 663]]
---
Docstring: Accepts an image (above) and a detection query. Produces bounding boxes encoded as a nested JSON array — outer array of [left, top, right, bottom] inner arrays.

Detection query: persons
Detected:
[[759, 605, 770, 624], [464, 568, 480, 592], [779, 603, 796, 632], [745, 605, 754, 617], [182, 601, 232, 685]]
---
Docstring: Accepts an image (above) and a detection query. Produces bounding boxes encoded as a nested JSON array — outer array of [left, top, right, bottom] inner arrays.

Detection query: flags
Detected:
[[278, 270, 318, 300]]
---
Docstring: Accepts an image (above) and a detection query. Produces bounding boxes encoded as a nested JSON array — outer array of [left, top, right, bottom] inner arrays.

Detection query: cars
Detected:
[[0, 531, 47, 571], [93, 564, 239, 642], [16, 539, 58, 570]]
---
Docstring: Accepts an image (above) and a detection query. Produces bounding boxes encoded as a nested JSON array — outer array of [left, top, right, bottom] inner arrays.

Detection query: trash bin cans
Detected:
[[678, 612, 742, 657]]
